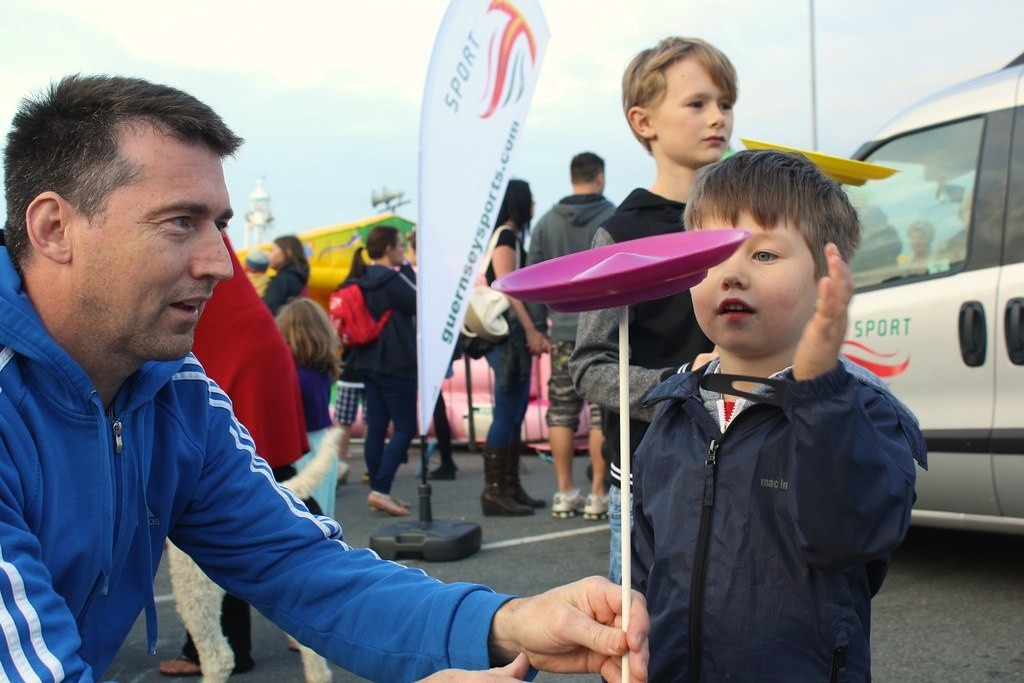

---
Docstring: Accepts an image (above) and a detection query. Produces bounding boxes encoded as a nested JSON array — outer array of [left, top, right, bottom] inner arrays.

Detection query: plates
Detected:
[[487, 226, 753, 314], [737, 136, 902, 187]]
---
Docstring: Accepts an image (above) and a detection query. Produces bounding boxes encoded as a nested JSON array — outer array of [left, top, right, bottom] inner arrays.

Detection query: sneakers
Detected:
[[551, 490, 586, 518], [583, 492, 610, 520]]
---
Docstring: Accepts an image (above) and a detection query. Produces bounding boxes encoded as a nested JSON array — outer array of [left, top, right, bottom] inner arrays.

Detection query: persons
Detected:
[[906, 189, 975, 269], [155, 225, 460, 676], [479, 151, 618, 522], [568, 34, 740, 584], [0, 70, 652, 683], [601, 147, 929, 683]]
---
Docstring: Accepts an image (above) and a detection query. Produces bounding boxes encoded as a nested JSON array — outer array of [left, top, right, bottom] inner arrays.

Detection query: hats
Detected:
[[246, 251, 269, 272]]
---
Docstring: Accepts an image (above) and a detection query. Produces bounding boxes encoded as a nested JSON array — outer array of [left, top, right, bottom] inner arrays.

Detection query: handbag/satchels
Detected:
[[460, 225, 522, 341]]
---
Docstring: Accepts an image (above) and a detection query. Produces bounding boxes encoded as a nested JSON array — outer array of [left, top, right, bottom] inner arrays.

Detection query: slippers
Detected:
[[160, 653, 256, 676]]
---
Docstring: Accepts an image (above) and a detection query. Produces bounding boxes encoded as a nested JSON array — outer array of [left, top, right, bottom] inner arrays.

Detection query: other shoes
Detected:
[[336, 460, 349, 484], [428, 465, 455, 480], [362, 473, 372, 481], [368, 494, 411, 516]]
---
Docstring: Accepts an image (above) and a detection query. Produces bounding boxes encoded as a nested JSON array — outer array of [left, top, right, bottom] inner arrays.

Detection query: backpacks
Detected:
[[327, 279, 392, 347]]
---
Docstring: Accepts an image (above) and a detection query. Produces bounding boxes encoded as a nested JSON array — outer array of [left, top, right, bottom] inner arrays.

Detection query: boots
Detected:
[[482, 446, 534, 516], [503, 442, 545, 509]]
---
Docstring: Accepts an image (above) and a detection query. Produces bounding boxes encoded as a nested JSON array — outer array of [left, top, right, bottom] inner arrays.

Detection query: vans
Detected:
[[770, 52, 1024, 540]]
